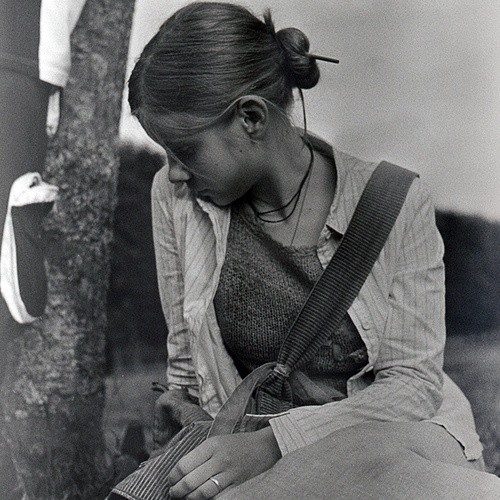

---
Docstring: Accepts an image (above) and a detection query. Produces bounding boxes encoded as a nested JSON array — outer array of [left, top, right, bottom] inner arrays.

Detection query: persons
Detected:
[[128, 2, 499, 500]]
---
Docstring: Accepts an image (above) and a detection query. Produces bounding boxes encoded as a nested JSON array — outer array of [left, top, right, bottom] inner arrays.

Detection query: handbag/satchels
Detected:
[[109, 363, 292, 500]]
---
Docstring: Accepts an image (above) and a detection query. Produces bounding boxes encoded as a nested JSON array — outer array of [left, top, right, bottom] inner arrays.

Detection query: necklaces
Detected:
[[246, 133, 313, 248]]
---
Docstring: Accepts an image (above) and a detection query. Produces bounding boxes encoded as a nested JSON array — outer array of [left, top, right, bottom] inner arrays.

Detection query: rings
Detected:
[[211, 477, 220, 492]]
